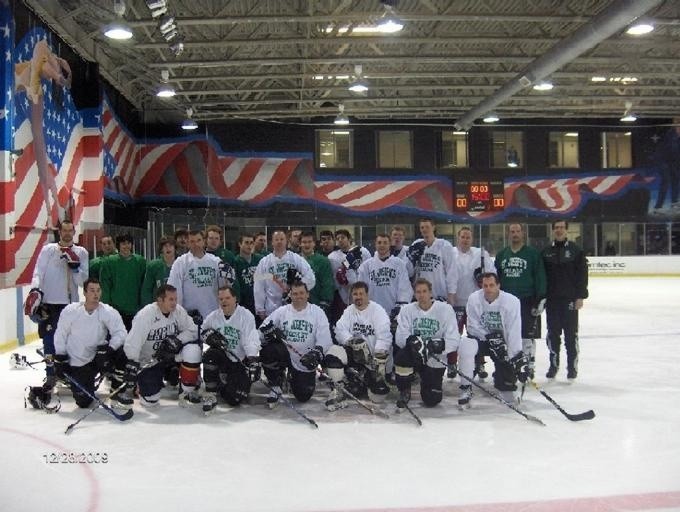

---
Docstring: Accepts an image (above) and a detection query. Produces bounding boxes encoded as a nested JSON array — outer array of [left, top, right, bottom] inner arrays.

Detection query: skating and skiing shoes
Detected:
[[397, 387, 411, 407]]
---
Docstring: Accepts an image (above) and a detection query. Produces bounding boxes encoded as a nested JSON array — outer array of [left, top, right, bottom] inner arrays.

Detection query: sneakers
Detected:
[[178, 386, 200, 404], [267, 385, 282, 402], [203, 398, 218, 411], [457, 385, 472, 404], [475, 356, 488, 378], [567, 366, 576, 377], [109, 388, 134, 405], [326, 388, 346, 404], [546, 365, 557, 377]]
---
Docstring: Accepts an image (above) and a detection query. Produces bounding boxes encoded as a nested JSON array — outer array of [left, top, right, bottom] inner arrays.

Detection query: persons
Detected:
[[23, 218, 588, 410], [605, 240, 617, 256]]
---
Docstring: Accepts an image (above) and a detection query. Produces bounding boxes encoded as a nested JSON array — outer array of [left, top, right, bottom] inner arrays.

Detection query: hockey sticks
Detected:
[[382, 375, 422, 426], [37, 349, 133, 421], [527, 376, 595, 421], [64, 360, 155, 435], [517, 316, 537, 404], [224, 348, 318, 428], [430, 352, 543, 426], [281, 337, 389, 419]]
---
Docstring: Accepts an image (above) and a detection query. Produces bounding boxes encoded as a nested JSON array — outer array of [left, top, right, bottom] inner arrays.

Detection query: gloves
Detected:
[[246, 356, 262, 383], [124, 359, 140, 383], [25, 288, 44, 316], [95, 345, 116, 372], [351, 337, 369, 363], [486, 333, 509, 363], [342, 246, 363, 270], [217, 262, 235, 284], [299, 346, 325, 369], [60, 247, 80, 269], [52, 354, 73, 379], [156, 335, 182, 365], [406, 241, 426, 262], [447, 363, 458, 378], [511, 351, 534, 383], [200, 328, 228, 351], [259, 320, 285, 342], [375, 353, 389, 375], [407, 335, 427, 367]]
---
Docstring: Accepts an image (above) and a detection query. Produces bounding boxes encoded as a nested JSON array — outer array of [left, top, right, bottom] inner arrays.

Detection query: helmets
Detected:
[[10, 353, 26, 368], [29, 387, 61, 413]]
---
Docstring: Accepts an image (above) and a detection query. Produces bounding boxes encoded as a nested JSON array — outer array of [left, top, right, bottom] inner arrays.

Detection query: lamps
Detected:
[[102, 0, 135, 41], [620, 101, 639, 123], [348, 65, 370, 93], [321, 140, 333, 157], [155, 69, 176, 98], [182, 108, 199, 130], [533, 76, 555, 90], [332, 105, 350, 125], [483, 108, 501, 123], [378, 0, 404, 34], [626, 17, 655, 35]]
